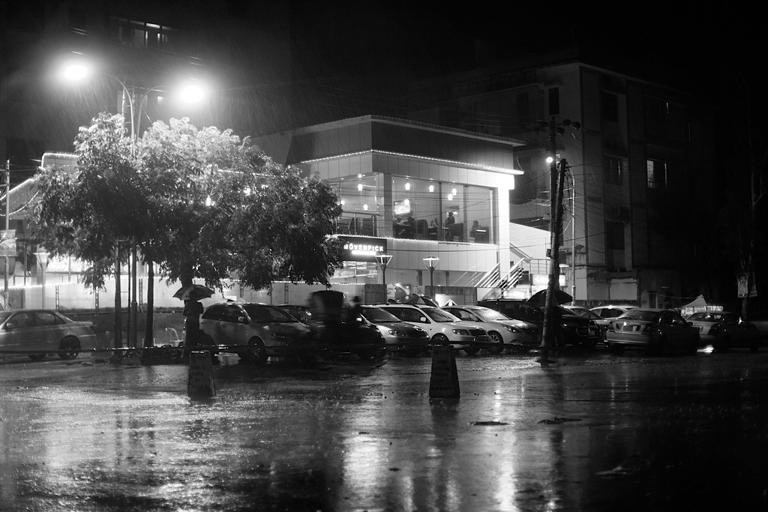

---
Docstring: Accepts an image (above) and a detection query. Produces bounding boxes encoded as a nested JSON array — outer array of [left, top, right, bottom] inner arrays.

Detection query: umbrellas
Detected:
[[524, 287, 572, 306], [174, 283, 215, 301]]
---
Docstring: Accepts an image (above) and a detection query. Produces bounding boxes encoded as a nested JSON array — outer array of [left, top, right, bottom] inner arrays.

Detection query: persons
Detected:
[[182, 304, 210, 356]]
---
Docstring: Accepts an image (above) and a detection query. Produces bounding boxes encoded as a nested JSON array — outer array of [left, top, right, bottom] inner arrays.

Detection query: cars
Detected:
[[0, 307, 98, 362]]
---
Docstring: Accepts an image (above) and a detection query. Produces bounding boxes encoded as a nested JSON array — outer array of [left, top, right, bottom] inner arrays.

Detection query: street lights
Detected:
[[61, 55, 213, 354], [421, 254, 439, 296], [653, 279, 673, 310], [545, 154, 577, 306], [557, 263, 570, 293], [376, 251, 393, 304]]
